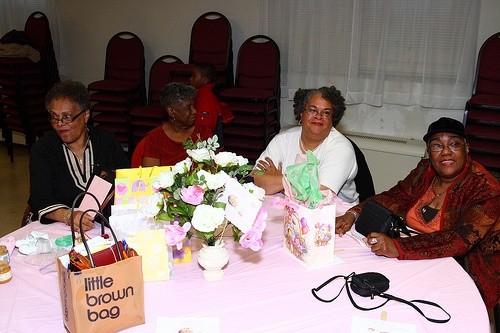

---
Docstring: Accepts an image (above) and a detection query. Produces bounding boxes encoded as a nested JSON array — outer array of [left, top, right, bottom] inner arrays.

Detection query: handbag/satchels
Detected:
[[355, 200, 412, 240], [281, 170, 336, 266], [349, 272, 390, 298], [58, 192, 145, 333]]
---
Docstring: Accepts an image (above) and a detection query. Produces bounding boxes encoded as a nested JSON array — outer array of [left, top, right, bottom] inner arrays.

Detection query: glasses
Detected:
[[429, 141, 466, 152], [304, 107, 334, 118], [48, 108, 87, 124]]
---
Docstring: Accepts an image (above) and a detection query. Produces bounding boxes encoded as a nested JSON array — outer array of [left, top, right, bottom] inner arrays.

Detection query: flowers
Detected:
[[151, 134, 269, 252]]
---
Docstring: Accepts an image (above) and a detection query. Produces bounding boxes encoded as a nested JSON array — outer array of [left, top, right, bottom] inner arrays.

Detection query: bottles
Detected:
[[0, 261, 13, 285], [0, 244, 11, 264]]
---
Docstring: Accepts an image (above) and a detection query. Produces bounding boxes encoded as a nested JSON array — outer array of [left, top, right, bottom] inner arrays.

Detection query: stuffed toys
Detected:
[[191, 81, 233, 145]]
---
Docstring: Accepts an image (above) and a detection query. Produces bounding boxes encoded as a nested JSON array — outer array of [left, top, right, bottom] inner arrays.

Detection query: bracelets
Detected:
[[64, 209, 72, 226], [346, 210, 358, 221]]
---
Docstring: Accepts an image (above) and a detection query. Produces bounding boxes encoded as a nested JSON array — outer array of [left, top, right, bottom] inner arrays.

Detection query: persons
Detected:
[[335, 117, 500, 333], [250, 86, 360, 218], [21, 80, 128, 235], [131, 83, 225, 168]]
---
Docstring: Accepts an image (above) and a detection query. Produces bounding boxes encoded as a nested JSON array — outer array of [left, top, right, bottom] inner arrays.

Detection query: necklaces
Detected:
[[300, 138, 324, 153], [68, 139, 88, 164]]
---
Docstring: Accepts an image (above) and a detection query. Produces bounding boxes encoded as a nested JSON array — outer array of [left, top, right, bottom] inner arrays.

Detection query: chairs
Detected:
[[87, 12, 281, 165], [0, 11, 60, 162], [462, 32, 500, 177]]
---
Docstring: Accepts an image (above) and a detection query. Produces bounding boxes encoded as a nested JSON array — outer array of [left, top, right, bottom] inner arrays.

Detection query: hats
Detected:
[[423, 118, 465, 143]]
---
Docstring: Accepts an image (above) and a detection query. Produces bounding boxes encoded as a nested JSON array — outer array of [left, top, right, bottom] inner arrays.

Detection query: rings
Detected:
[[371, 238, 377, 244], [264, 168, 267, 172]]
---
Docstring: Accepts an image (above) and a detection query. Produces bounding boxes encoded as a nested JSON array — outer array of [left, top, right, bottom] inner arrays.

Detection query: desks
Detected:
[[0, 192, 492, 333]]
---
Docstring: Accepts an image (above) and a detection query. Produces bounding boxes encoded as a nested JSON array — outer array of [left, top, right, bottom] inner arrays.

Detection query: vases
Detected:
[[197, 241, 229, 281]]
